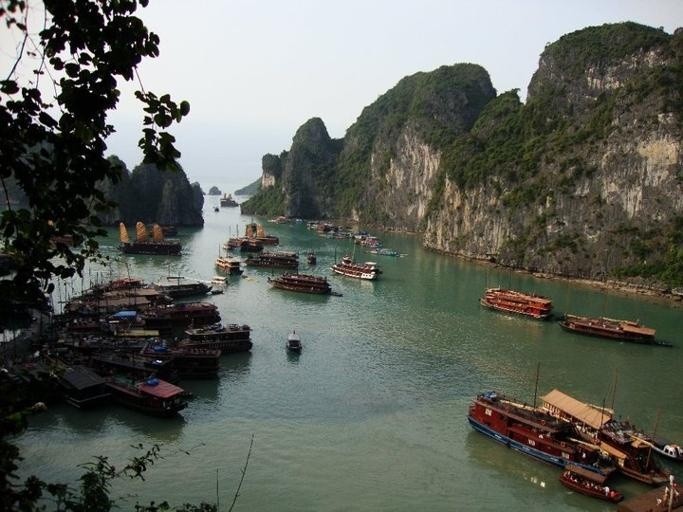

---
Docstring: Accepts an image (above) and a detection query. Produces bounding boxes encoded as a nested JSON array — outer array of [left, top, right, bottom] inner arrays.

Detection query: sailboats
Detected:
[[222, 223, 280, 253], [118, 222, 182, 255]]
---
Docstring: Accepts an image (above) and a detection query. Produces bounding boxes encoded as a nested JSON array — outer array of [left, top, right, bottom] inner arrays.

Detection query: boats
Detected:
[[558, 313, 656, 344], [0, 274, 253, 420], [219, 193, 238, 208], [265, 273, 331, 295], [477, 287, 553, 321], [329, 255, 384, 282], [244, 251, 299, 270], [466, 360, 682, 511], [214, 256, 242, 276], [287, 330, 302, 354], [307, 253, 315, 265], [305, 221, 400, 256]]
